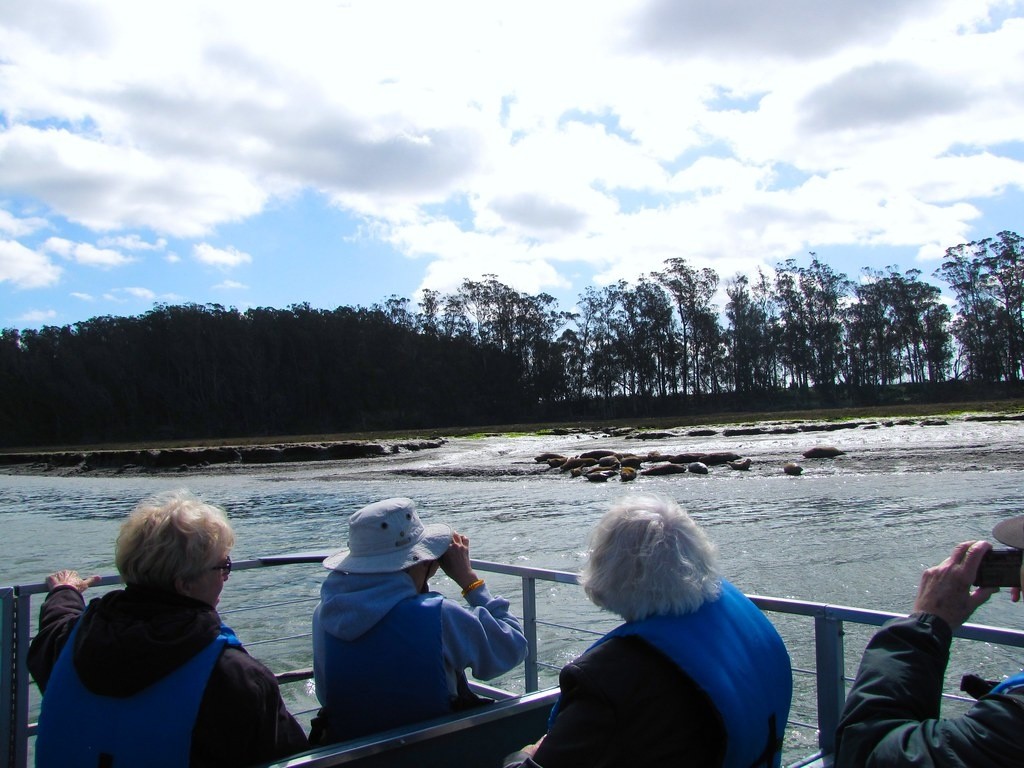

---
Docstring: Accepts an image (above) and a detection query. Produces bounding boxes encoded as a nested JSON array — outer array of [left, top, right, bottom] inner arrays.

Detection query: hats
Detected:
[[992, 514, 1024, 550], [323, 497, 453, 573]]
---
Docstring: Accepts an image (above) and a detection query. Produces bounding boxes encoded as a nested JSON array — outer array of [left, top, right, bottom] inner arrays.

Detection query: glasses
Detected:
[[212, 556, 231, 575]]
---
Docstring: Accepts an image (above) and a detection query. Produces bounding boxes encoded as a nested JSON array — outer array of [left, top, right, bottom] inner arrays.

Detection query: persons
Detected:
[[306, 498, 529, 752], [26, 491, 306, 768], [504, 491, 794, 768], [834, 513, 1024, 768]]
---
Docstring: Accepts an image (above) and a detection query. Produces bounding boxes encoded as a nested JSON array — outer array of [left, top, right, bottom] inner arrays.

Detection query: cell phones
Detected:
[[973, 547, 1023, 587]]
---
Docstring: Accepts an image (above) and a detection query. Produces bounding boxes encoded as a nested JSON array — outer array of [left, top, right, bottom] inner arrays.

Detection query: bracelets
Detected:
[[461, 578, 484, 598]]
